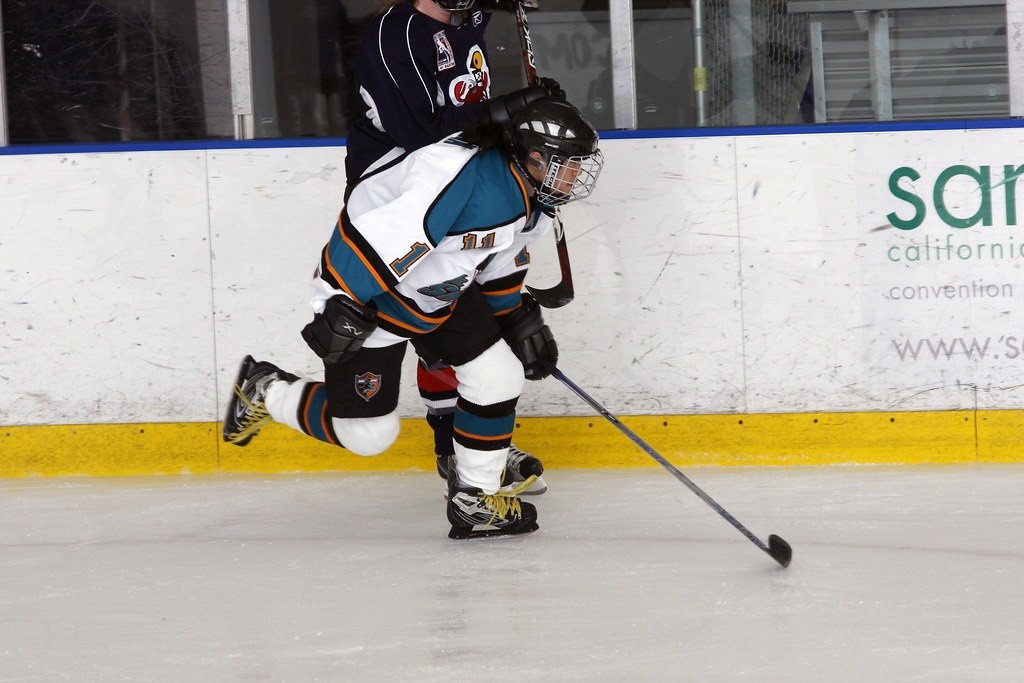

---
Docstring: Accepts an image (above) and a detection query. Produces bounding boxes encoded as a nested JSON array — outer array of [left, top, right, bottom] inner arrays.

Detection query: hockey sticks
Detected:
[[550, 365, 793, 569], [509, 0, 578, 312]]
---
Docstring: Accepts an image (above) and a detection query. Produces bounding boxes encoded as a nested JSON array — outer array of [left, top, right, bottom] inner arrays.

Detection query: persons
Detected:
[[222, 0, 603, 541]]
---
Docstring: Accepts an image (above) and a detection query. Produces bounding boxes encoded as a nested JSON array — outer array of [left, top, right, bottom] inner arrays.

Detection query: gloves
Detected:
[[301, 295, 378, 367], [487, 77, 566, 128], [496, 293, 558, 380]]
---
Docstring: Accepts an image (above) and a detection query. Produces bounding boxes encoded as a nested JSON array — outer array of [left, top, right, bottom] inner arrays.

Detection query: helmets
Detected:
[[510, 96, 599, 206]]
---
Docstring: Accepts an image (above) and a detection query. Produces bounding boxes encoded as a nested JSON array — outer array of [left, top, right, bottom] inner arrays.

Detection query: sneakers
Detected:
[[436, 442, 548, 500], [447, 467, 539, 539], [223, 354, 301, 447]]
